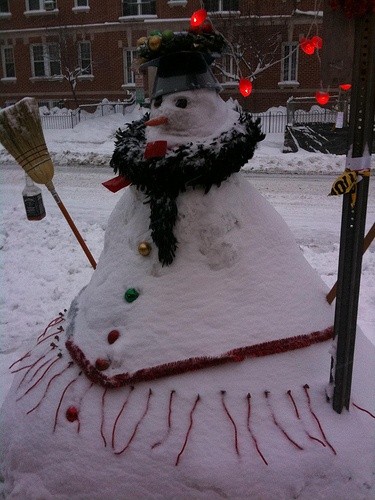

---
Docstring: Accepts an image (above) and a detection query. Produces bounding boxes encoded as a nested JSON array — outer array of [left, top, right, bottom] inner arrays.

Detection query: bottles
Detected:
[[22, 171, 46, 221]]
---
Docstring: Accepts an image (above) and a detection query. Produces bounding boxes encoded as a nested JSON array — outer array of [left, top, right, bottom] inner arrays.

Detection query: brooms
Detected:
[[1, 96, 97, 270]]
